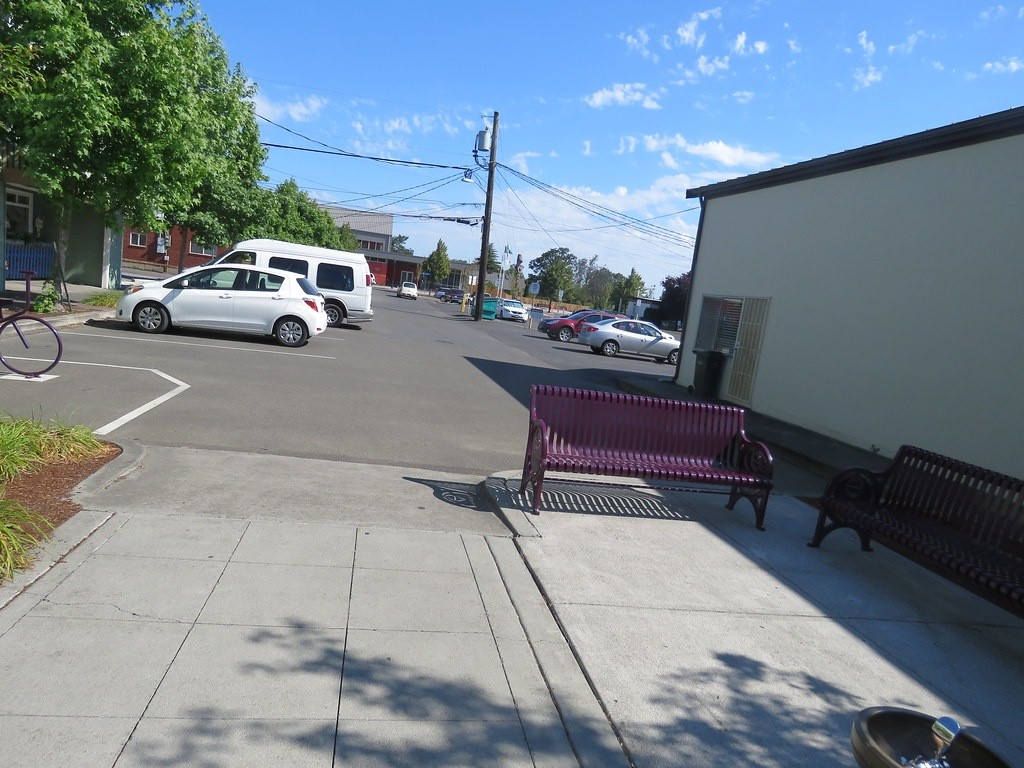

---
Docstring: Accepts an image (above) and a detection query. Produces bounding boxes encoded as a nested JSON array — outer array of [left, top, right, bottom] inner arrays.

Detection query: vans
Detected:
[[181, 238, 374, 327]]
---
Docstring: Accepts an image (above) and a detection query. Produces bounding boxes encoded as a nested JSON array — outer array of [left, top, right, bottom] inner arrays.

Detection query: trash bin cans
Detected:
[[688, 349, 733, 401], [471, 298, 498, 320]]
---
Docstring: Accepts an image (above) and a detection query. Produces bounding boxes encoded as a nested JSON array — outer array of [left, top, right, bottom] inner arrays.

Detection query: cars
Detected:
[[496, 298, 529, 322], [397, 282, 419, 300], [578, 317, 680, 366], [116, 264, 328, 347], [468, 291, 490, 306], [434, 287, 452, 302], [371, 273, 376, 285], [538, 309, 635, 343], [446, 289, 465, 303]]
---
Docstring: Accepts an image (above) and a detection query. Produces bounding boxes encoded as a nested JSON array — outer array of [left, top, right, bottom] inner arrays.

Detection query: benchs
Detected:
[[519, 384, 774, 531], [806, 444, 1024, 619]]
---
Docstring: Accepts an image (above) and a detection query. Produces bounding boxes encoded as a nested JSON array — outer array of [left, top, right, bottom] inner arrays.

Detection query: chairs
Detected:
[[260, 278, 265, 291], [624, 324, 638, 332]]
